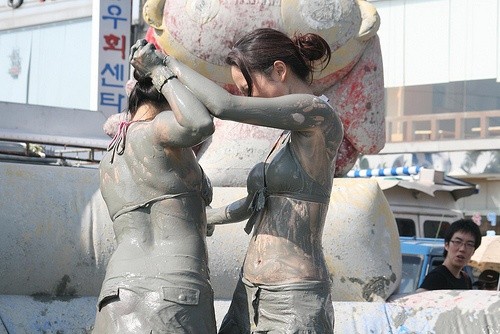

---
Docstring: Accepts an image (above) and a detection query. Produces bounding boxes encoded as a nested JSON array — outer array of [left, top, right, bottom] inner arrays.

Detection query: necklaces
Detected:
[[281, 129, 290, 144]]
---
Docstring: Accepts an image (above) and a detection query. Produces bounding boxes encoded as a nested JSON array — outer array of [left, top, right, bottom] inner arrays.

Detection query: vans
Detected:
[[373, 166, 476, 239]]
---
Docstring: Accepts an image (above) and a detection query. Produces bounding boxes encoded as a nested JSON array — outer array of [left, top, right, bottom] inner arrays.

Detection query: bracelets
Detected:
[[162, 54, 170, 65], [153, 72, 177, 92]]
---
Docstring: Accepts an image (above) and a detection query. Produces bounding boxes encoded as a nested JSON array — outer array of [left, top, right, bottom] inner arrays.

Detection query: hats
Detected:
[[472, 270, 500, 285]]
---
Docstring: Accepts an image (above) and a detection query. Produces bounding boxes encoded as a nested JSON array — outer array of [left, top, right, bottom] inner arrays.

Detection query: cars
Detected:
[[386, 237, 499, 303]]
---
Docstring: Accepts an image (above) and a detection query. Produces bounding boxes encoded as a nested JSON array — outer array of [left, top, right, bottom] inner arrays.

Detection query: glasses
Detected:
[[450, 240, 475, 249], [477, 283, 498, 290]]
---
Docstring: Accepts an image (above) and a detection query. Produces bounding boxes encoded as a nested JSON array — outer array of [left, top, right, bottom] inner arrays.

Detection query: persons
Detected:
[[416, 219, 482, 294], [129, 28, 344, 334], [471, 269, 500, 291], [96, 39, 217, 334]]
